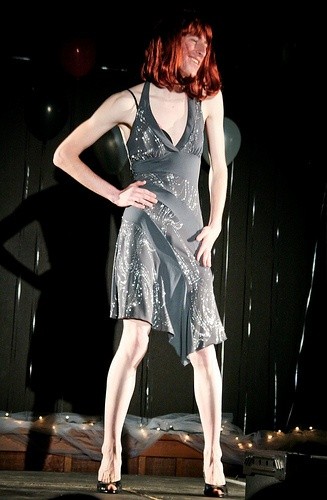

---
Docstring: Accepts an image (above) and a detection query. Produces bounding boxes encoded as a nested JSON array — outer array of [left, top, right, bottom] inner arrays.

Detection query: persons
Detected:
[[52, 11, 229, 497]]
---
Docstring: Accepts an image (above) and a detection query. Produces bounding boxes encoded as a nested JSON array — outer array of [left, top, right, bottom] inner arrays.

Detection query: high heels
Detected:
[[202, 455, 227, 499], [97, 479, 122, 494]]
